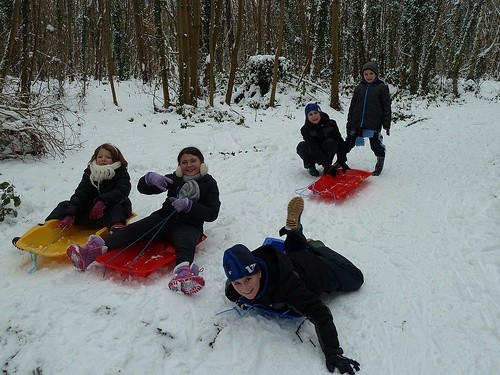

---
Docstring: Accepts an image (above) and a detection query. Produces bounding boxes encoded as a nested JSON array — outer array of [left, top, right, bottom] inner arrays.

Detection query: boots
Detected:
[[64, 235, 106, 271], [169, 261, 206, 294]]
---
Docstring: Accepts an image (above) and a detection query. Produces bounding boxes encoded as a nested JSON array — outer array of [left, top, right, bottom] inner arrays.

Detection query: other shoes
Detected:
[[111, 223, 125, 235], [308, 238, 326, 249], [309, 164, 321, 177], [285, 195, 304, 232], [11, 235, 27, 251], [371, 162, 383, 176]]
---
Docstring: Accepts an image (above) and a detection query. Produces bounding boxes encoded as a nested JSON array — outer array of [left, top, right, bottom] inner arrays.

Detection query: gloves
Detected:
[[385, 129, 391, 136], [328, 166, 339, 177], [90, 200, 106, 219], [340, 163, 351, 174], [170, 196, 193, 212], [325, 355, 362, 375], [145, 171, 173, 191], [59, 214, 76, 229]]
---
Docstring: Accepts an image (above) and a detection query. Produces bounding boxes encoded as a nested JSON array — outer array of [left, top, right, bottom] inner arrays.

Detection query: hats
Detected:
[[363, 61, 380, 76], [223, 242, 262, 279], [305, 101, 323, 114]]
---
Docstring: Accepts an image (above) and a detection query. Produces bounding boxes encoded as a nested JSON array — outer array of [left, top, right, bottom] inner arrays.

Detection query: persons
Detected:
[[12, 143, 132, 251], [223, 198, 364, 375], [334, 62, 391, 176], [68, 148, 220, 295], [296, 102, 351, 177]]
